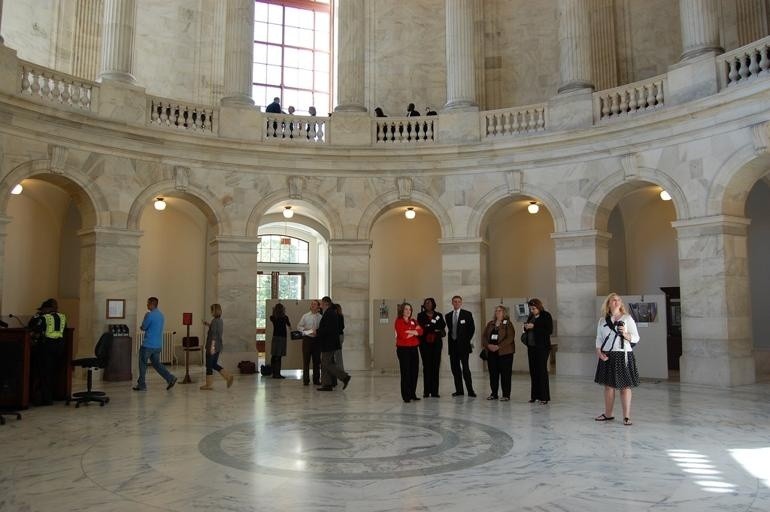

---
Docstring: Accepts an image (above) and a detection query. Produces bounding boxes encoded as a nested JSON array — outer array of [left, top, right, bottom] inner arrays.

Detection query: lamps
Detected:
[[405, 208, 416, 219], [154, 198, 166, 211], [659, 190, 672, 201], [528, 202, 540, 214], [283, 206, 293, 218], [10, 183, 23, 195]]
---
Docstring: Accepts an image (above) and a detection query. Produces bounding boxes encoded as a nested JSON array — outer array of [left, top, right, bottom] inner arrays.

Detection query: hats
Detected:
[[38, 298, 57, 309]]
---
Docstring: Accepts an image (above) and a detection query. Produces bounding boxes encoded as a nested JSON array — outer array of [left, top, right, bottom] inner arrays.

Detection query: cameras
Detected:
[[613, 321, 625, 326]]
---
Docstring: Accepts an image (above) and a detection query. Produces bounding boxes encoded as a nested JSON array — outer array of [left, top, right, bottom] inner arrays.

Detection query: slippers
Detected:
[[624, 417, 632, 425], [595, 414, 615, 420]]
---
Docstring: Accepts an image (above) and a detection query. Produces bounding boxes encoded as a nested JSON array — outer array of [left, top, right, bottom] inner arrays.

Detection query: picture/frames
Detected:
[[106, 299, 126, 319]]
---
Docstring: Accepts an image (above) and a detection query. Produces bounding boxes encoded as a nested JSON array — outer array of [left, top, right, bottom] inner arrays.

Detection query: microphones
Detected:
[[8, 313, 29, 328]]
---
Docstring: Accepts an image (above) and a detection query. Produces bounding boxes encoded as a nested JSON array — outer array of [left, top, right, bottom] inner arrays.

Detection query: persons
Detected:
[[394, 302, 424, 403], [481, 305, 516, 403], [269, 302, 292, 381], [131, 295, 177, 390], [445, 295, 478, 398], [264, 96, 437, 142], [296, 295, 352, 392], [416, 298, 446, 399], [28, 298, 69, 407], [520, 298, 553, 406], [592, 290, 642, 426], [200, 303, 234, 391]]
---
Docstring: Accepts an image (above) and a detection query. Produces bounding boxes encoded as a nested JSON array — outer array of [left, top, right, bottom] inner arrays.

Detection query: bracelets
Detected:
[[210, 346, 215, 350]]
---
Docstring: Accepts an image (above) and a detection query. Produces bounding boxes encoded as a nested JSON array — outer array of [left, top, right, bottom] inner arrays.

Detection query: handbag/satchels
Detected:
[[521, 332, 528, 346], [240, 363, 255, 374], [480, 349, 488, 360], [302, 335, 324, 352]]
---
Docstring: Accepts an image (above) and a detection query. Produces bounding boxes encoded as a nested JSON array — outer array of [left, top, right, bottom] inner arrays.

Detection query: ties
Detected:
[[452, 312, 457, 340]]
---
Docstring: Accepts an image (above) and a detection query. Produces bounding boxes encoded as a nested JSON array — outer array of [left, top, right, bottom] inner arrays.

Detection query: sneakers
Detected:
[[272, 374, 286, 379], [304, 380, 321, 385], [133, 387, 147, 391], [318, 386, 332, 391], [343, 375, 351, 390], [167, 376, 177, 390]]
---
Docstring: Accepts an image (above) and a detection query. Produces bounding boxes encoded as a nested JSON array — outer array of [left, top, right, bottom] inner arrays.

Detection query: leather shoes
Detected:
[[423, 392, 440, 398], [487, 395, 495, 400], [528, 398, 547, 405], [405, 396, 420, 402], [500, 397, 510, 401]]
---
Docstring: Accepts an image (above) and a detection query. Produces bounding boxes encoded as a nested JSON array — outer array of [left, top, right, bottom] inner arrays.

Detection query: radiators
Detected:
[[137, 331, 176, 365]]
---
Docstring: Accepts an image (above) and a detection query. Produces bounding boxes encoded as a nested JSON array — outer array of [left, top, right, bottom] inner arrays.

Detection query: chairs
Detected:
[[65, 332, 113, 408], [0, 383, 28, 425]]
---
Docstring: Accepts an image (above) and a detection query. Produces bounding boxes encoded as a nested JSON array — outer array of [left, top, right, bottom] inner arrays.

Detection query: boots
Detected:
[[219, 368, 234, 388], [200, 375, 214, 390]]
